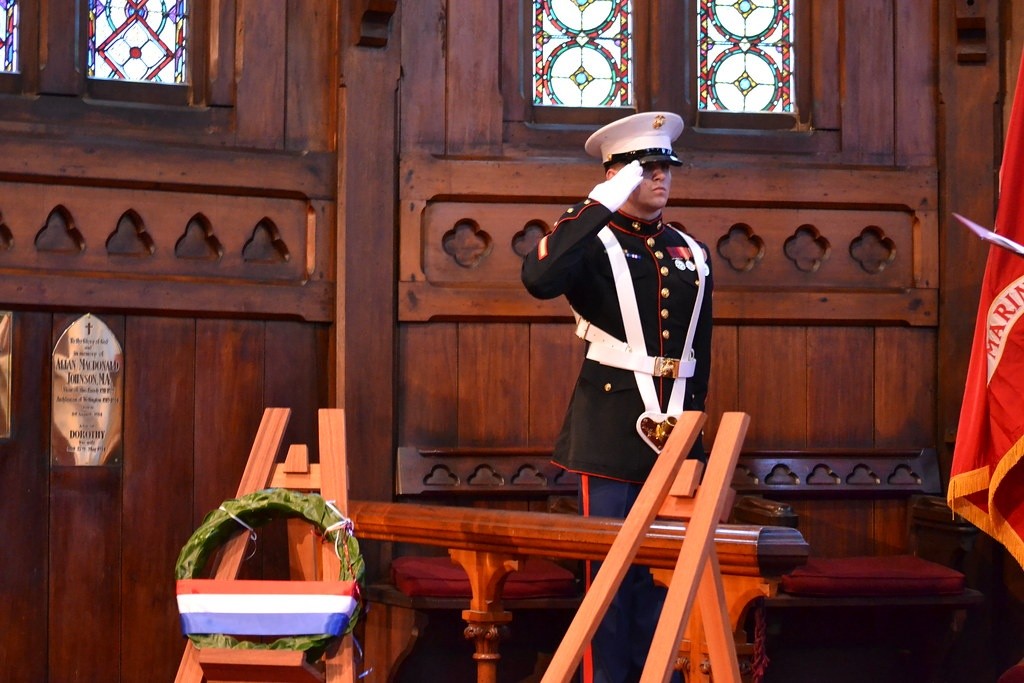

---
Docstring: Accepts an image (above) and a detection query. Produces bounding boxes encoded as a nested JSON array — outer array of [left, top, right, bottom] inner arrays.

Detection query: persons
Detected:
[[520, 111, 714, 683]]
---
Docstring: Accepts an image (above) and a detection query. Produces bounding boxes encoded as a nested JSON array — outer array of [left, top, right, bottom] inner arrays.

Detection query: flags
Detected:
[[946, 44, 1024, 572]]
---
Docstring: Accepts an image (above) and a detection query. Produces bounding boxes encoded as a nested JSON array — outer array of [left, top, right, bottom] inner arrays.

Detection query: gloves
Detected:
[[586, 160, 644, 213]]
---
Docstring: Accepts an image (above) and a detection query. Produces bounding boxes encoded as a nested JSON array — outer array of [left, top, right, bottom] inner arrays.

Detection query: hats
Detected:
[[584, 110, 686, 167]]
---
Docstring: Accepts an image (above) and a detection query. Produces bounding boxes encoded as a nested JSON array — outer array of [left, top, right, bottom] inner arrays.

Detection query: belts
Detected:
[[571, 307, 697, 379]]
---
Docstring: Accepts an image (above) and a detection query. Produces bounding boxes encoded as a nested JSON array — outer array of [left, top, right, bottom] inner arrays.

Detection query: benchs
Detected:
[[360, 554, 986, 683]]
